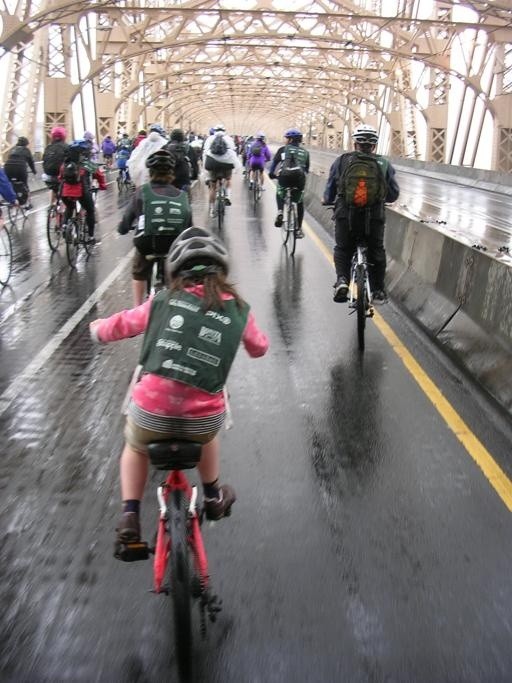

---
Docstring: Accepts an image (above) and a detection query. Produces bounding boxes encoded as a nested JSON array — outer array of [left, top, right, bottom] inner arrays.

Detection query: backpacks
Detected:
[[43, 143, 68, 176], [251, 143, 265, 156], [63, 161, 85, 183], [337, 152, 386, 208], [207, 134, 229, 155], [279, 154, 305, 188]]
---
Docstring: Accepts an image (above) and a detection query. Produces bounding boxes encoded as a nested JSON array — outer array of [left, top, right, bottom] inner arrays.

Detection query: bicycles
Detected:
[[64, 187, 103, 267], [267, 173, 301, 256], [250, 170, 264, 202], [44, 183, 67, 252], [105, 153, 134, 194], [7, 171, 37, 225], [339, 244, 378, 351], [212, 173, 229, 230], [116, 438, 222, 676], [0, 203, 14, 284]]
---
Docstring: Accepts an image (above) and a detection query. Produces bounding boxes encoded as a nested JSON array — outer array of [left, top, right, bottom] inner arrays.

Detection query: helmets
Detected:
[[146, 149, 177, 171], [163, 225, 231, 286], [285, 129, 302, 138], [17, 136, 29, 146], [171, 129, 186, 141], [351, 124, 379, 145], [255, 134, 264, 141], [51, 127, 65, 142], [150, 124, 161, 133], [213, 124, 225, 131]]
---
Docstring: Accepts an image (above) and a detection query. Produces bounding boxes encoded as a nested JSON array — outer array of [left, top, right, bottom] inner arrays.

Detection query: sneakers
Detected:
[[372, 288, 389, 306], [202, 483, 236, 520], [224, 194, 231, 206], [295, 228, 305, 239], [332, 276, 350, 304], [114, 510, 141, 560], [209, 208, 217, 218], [275, 209, 283, 227]]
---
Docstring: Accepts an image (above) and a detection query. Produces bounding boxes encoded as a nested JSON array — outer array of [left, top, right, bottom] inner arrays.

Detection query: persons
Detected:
[[87, 224, 271, 549], [320, 124, 400, 305], [186, 123, 272, 218], [268, 128, 310, 239], [3, 135, 37, 211], [159, 127, 199, 207], [116, 149, 193, 308], [41, 122, 171, 245], [0, 165, 19, 233]]
